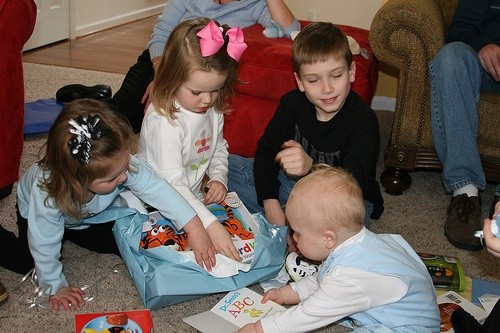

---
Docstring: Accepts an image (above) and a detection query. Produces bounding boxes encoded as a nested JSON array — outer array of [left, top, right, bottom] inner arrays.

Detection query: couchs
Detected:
[[370, 0, 500, 195], [0, 0, 37, 200]]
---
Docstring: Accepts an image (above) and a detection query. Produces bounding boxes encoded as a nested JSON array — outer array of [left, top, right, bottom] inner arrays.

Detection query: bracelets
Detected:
[[153, 61, 159, 67]]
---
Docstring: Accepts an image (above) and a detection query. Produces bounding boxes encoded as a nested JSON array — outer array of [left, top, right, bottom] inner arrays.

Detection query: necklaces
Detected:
[[219, 1, 223, 4]]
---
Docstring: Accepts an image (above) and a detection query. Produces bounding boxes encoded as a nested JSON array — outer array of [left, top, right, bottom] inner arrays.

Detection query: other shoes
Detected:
[[445, 193, 486, 250], [286, 252, 318, 282]]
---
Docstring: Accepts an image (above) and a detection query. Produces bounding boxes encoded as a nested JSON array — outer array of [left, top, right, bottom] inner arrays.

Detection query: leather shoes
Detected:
[[56, 83, 112, 102]]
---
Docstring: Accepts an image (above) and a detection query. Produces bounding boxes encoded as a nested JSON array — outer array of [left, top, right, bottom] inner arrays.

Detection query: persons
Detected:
[[56, 0, 301, 137], [234, 163, 441, 333], [0, 98, 217, 312], [253, 22, 385, 260], [429, 0, 500, 251], [134, 16, 248, 263], [0, 0, 37, 306], [450, 201, 500, 333]]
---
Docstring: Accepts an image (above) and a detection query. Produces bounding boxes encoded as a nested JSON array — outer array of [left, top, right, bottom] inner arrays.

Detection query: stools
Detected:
[[219, 19, 380, 160]]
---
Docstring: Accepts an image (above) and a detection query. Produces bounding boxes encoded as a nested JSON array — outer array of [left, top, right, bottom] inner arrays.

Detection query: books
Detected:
[[75, 309, 154, 333], [437, 291, 488, 333], [182, 287, 286, 333]]
